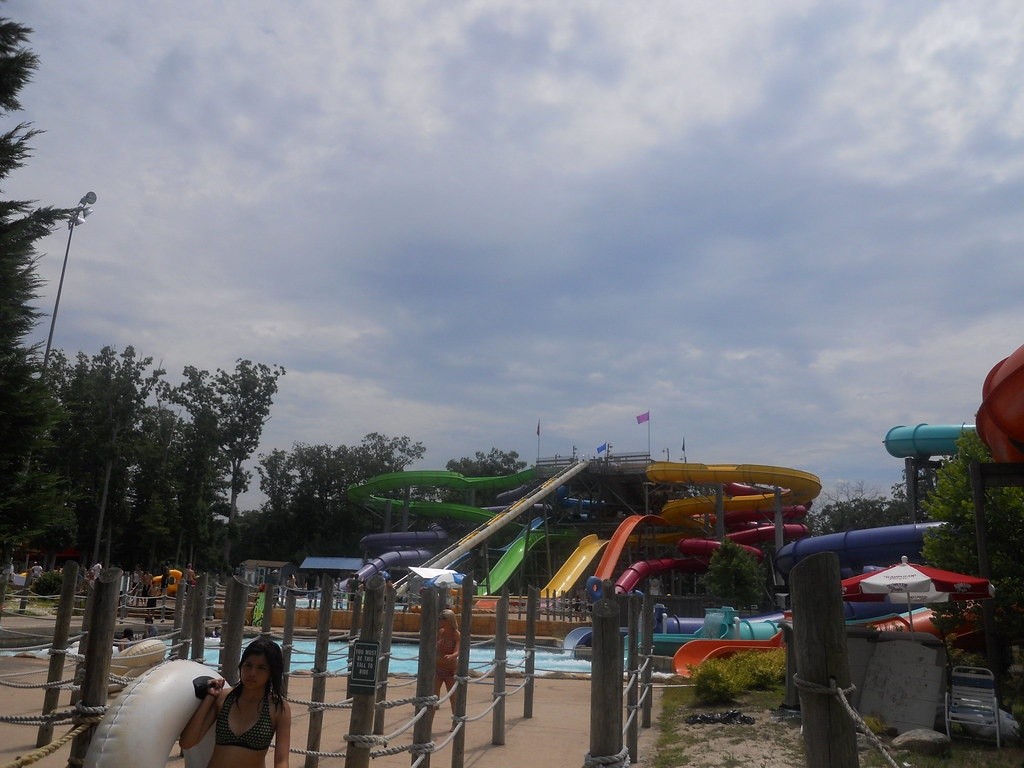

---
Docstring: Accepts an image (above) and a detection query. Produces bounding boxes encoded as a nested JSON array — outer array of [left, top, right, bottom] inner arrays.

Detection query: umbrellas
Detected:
[[840, 556, 996, 632], [424, 572, 478, 609]]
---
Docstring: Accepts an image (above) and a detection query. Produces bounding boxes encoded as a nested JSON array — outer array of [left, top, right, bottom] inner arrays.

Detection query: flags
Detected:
[[682, 438, 685, 450], [537, 420, 540, 435], [636, 412, 649, 424], [597, 444, 606, 453]]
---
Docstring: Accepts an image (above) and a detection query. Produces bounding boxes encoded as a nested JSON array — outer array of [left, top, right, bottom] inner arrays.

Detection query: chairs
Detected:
[[943, 666, 1000, 747]]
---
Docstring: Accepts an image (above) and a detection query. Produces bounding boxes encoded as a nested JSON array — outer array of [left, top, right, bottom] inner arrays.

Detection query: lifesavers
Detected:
[[82, 659, 232, 768], [109, 640, 166, 677]]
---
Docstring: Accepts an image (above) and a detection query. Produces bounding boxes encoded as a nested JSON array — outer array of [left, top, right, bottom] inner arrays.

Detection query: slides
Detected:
[[333, 343, 1024, 680]]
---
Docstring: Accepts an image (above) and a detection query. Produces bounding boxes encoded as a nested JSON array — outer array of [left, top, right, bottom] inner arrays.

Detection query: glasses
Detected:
[[439, 616, 447, 620]]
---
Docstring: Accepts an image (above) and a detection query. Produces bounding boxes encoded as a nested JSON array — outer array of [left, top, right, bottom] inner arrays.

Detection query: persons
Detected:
[[161, 560, 171, 595], [434, 609, 460, 732], [2, 556, 14, 583], [347, 573, 366, 608], [148, 581, 160, 607], [185, 563, 220, 592], [270, 570, 280, 604], [133, 561, 153, 596], [304, 571, 319, 609], [378, 571, 383, 576], [142, 616, 159, 639], [118, 629, 133, 652], [286, 574, 298, 588], [177, 638, 291, 768], [94, 560, 102, 577], [29, 561, 43, 585]]
